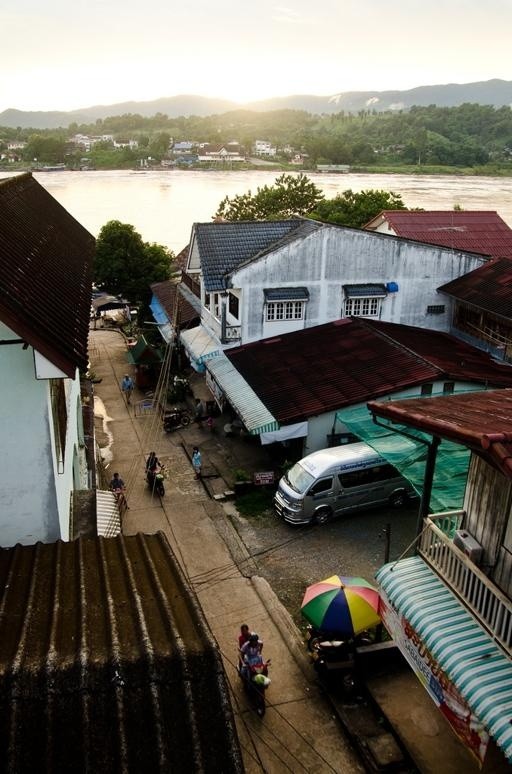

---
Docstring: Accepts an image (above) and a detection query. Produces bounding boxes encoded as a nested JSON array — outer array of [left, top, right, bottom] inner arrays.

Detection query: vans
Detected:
[[272, 435, 424, 528]]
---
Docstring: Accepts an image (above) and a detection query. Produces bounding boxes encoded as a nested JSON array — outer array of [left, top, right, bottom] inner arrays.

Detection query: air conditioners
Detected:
[[452, 530, 483, 569]]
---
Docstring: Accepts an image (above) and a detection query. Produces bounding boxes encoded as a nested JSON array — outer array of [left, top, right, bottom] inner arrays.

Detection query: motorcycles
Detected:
[[236, 652, 272, 718], [110, 484, 127, 513], [164, 408, 191, 433], [144, 464, 166, 497]]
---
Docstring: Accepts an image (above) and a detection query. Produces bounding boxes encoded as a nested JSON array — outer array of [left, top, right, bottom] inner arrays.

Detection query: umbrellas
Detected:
[[300, 574, 382, 640]]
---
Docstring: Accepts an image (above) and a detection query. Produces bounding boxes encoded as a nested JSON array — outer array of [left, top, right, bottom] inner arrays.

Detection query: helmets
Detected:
[[248, 633, 258, 641]]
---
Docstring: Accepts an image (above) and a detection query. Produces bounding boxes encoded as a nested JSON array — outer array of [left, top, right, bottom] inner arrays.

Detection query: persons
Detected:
[[208, 416, 216, 433], [121, 374, 134, 404], [192, 447, 201, 481], [145, 452, 164, 488], [239, 632, 263, 685], [236, 624, 254, 670], [194, 399, 204, 429], [109, 473, 130, 510]]
